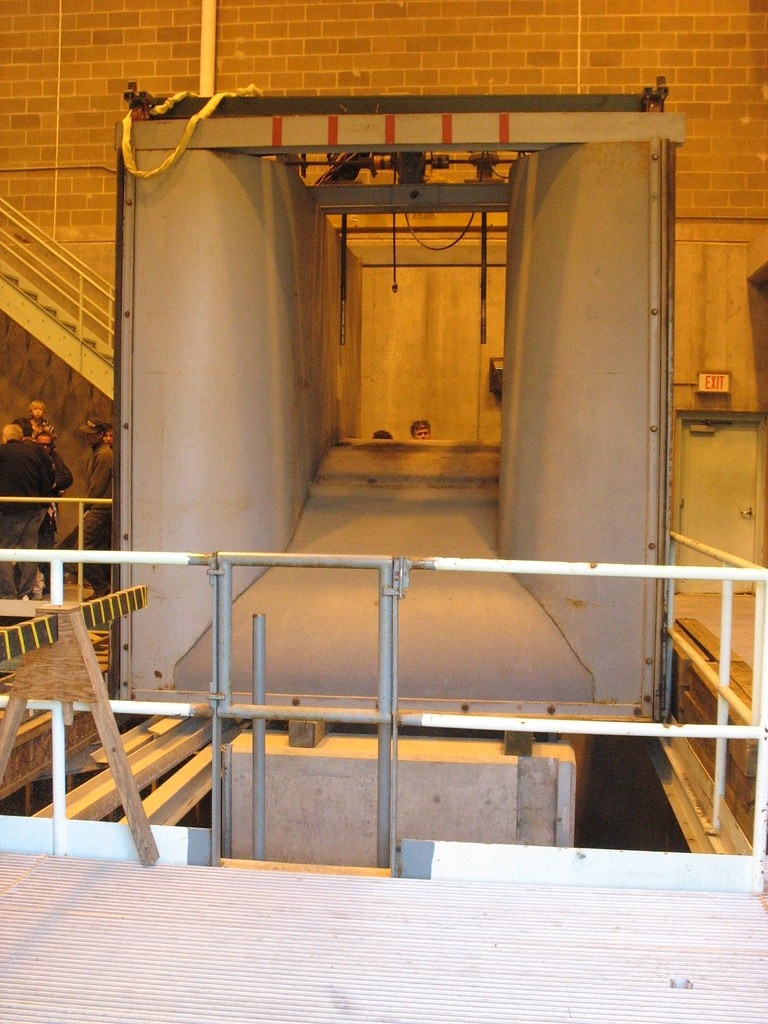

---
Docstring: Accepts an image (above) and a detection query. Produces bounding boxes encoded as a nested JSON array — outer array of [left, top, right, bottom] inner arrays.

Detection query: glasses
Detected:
[[37, 442, 50, 447]]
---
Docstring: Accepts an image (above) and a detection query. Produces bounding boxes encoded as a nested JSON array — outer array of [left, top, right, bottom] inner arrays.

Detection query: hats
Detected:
[[78, 419, 109, 434]]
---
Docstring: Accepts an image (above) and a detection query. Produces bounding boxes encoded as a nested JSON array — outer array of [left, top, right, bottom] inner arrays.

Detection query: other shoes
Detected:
[[33, 591, 44, 600], [82, 586, 110, 602]]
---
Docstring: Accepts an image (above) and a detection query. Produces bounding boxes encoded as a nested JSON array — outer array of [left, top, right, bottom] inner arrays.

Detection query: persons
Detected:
[[0, 399, 113, 600], [410, 420, 431, 440]]
